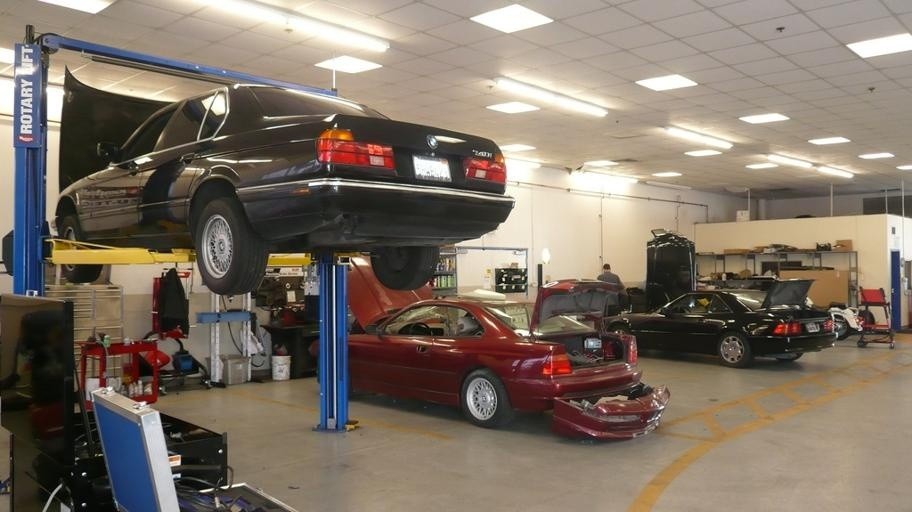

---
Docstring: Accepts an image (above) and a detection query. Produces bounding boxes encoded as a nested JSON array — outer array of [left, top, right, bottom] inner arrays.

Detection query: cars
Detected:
[[605, 275, 838, 369], [54, 84, 515, 296], [309, 264, 672, 440]]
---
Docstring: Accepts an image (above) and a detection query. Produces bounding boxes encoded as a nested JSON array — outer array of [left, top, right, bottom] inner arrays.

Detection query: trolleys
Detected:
[[856, 285, 896, 349]]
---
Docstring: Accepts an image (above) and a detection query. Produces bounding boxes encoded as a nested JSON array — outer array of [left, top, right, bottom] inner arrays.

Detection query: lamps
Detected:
[[662, 124, 734, 150], [817, 165, 855, 179], [766, 153, 813, 169], [195, 0, 390, 54], [492, 73, 609, 118]]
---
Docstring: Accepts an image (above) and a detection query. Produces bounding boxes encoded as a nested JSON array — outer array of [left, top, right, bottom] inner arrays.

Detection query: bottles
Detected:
[[722, 272, 726, 281], [129, 380, 143, 398], [104, 335, 110, 348]]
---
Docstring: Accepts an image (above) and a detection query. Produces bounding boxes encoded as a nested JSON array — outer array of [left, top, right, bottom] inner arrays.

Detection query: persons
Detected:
[[597, 263, 626, 316]]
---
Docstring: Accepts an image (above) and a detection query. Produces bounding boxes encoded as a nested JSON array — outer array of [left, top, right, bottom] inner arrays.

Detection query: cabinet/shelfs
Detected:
[[260, 319, 319, 379], [431, 245, 458, 297], [695, 248, 859, 308]]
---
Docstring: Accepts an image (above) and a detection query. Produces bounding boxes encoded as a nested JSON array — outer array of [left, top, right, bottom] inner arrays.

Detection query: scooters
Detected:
[[805, 296, 866, 341]]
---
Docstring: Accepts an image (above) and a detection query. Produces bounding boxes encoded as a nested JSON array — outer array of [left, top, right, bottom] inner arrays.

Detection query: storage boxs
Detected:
[[204, 353, 248, 385]]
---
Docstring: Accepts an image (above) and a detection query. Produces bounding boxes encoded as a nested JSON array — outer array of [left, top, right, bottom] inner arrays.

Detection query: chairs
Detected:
[[458, 315, 479, 336], [443, 316, 457, 335]]
